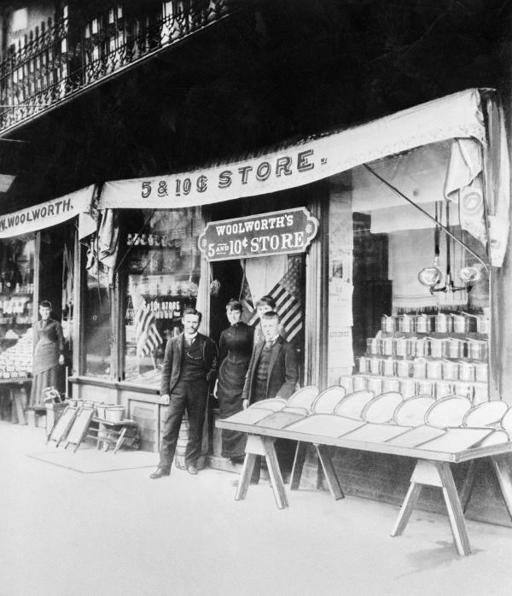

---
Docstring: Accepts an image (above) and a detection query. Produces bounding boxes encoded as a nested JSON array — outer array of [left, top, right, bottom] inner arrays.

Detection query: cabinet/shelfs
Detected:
[[0, 377, 32, 425], [213, 410, 512, 558]]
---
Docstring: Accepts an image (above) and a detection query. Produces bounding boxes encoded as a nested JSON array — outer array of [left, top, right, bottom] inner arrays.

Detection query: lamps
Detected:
[[418, 201, 484, 294]]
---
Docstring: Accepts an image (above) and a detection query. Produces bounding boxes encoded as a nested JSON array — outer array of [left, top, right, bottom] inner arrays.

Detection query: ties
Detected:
[[187, 338, 193, 348]]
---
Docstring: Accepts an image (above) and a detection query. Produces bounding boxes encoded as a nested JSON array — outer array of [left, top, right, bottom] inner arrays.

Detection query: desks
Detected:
[[87, 418, 137, 453]]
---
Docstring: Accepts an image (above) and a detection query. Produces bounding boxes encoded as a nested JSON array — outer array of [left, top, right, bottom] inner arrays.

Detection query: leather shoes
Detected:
[[187, 466, 197, 474], [150, 468, 171, 479]]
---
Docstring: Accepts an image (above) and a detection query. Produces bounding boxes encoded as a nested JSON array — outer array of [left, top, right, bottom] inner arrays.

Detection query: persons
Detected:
[[213, 299, 255, 463], [242, 312, 298, 410], [252, 295, 285, 352], [151, 308, 218, 478], [28, 300, 64, 416]]
